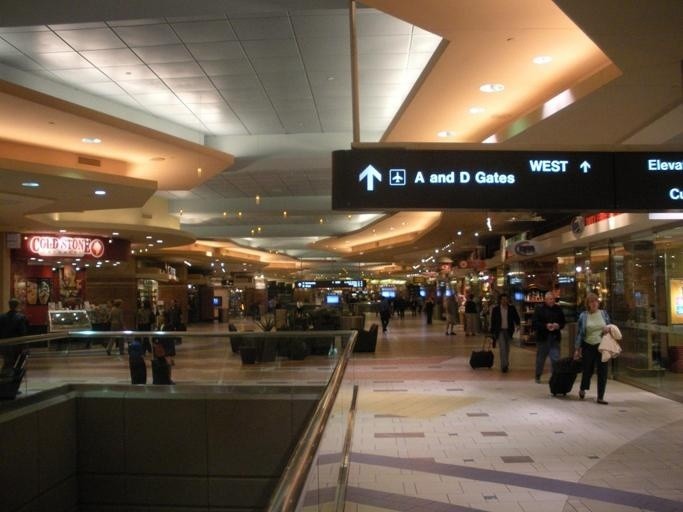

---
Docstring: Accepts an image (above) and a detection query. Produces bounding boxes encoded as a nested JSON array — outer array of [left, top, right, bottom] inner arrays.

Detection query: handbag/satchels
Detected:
[[153, 340, 167, 359], [129, 342, 144, 358]]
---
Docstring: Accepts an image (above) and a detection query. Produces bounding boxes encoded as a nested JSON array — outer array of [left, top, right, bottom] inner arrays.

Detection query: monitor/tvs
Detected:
[[213, 296, 221, 307], [326, 296, 340, 304], [418, 288, 525, 301], [383, 289, 396, 298]]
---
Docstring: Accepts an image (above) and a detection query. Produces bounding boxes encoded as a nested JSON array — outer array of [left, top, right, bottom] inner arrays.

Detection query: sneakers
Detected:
[[579, 390, 585, 398], [597, 399, 608, 404], [501, 367, 508, 373], [534, 376, 541, 383]]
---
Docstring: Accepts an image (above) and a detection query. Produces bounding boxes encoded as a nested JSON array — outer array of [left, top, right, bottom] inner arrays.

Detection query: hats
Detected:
[[9, 297, 20, 305]]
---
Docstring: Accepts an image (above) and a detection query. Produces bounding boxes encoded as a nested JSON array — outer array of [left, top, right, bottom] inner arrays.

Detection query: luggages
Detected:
[[127, 338, 146, 384], [0, 353, 29, 401], [469, 335, 495, 370], [549, 356, 578, 398], [151, 337, 172, 384]]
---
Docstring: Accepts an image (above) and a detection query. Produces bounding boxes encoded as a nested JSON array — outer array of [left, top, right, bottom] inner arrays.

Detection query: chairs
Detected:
[[229, 320, 378, 361]]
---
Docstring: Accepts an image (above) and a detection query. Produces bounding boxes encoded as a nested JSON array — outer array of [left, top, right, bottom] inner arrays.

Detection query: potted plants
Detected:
[[252, 313, 280, 361], [239, 331, 257, 363]]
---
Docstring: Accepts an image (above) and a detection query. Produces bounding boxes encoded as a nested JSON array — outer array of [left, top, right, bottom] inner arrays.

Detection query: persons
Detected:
[[531, 291, 566, 384], [106, 297, 182, 384], [573, 293, 612, 406], [463, 294, 477, 336], [445, 295, 457, 336], [488, 298, 499, 349], [424, 297, 434, 325], [0, 296, 31, 396], [388, 295, 424, 320], [490, 293, 522, 373], [380, 298, 388, 331]]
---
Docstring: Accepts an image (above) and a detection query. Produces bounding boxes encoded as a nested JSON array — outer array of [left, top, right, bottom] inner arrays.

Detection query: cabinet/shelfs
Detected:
[[48, 309, 93, 332], [521, 284, 549, 345]]
